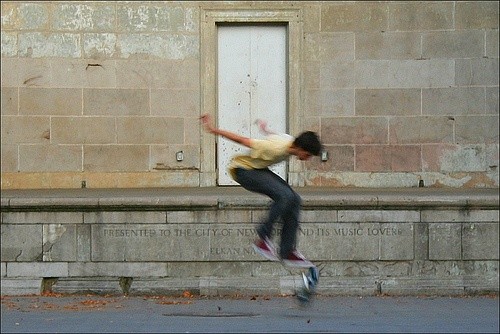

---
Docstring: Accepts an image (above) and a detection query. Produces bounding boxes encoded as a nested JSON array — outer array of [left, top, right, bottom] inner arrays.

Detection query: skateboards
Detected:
[[298, 267, 318, 307]]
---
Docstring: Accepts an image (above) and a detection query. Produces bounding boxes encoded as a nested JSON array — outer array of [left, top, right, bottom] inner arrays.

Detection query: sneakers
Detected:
[[283, 251, 316, 267], [253, 239, 280, 261]]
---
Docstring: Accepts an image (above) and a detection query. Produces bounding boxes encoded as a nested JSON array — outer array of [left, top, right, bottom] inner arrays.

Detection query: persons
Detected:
[[195, 112, 322, 271]]
